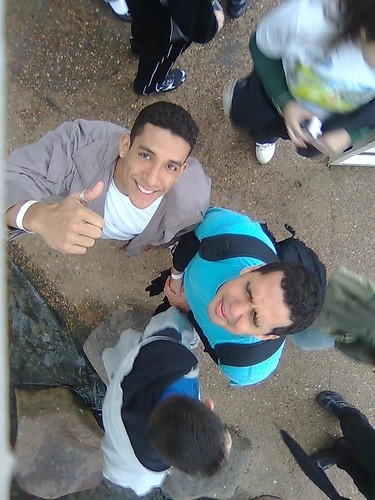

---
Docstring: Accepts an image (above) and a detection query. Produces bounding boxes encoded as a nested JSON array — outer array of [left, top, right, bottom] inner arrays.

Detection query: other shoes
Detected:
[[157, 68, 187, 92], [254, 141, 276, 163]]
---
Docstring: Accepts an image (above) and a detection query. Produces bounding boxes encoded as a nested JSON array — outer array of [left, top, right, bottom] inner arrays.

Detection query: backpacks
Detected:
[[275, 232, 327, 330]]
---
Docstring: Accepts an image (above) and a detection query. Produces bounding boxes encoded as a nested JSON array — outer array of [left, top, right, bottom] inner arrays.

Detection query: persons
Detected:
[[100, 0, 246, 95], [1, 101, 211, 257], [143, 205, 374, 386], [308, 391, 375, 500], [223, 0, 374, 165], [100, 294, 233, 496]]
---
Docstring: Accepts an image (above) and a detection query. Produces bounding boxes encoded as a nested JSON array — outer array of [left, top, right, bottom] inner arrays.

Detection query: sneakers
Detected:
[[309, 448, 336, 471], [316, 390, 355, 418]]
